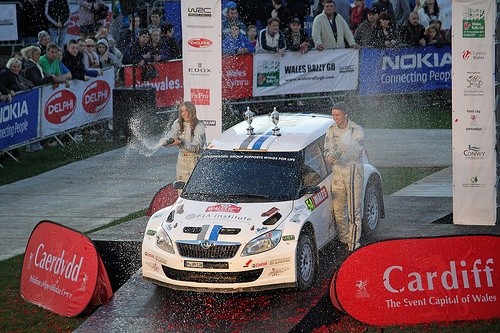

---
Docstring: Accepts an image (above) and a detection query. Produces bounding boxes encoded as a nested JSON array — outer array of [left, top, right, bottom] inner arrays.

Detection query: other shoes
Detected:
[[74, 133, 83, 141], [90, 130, 99, 134], [47, 137, 61, 145], [333, 241, 348, 252], [26, 142, 43, 152]]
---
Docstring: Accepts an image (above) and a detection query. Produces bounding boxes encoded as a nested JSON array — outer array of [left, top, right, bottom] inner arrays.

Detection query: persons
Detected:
[[221, 0, 452, 119], [162, 102, 206, 196], [324, 101, 365, 254], [0, 0, 182, 158]]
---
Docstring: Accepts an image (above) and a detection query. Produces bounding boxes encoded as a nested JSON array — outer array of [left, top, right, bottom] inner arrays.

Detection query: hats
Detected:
[[291, 18, 301, 24], [369, 7, 379, 14]]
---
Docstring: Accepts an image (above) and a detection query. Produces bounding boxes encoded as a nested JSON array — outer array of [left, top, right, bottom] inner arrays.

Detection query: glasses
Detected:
[[87, 44, 95, 47]]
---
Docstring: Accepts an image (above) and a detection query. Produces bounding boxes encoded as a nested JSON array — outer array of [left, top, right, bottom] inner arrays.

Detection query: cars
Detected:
[[142, 106, 385, 294]]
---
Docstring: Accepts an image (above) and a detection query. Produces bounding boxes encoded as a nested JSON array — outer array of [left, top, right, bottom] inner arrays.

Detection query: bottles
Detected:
[[331, 147, 341, 166], [166, 135, 180, 144]]
